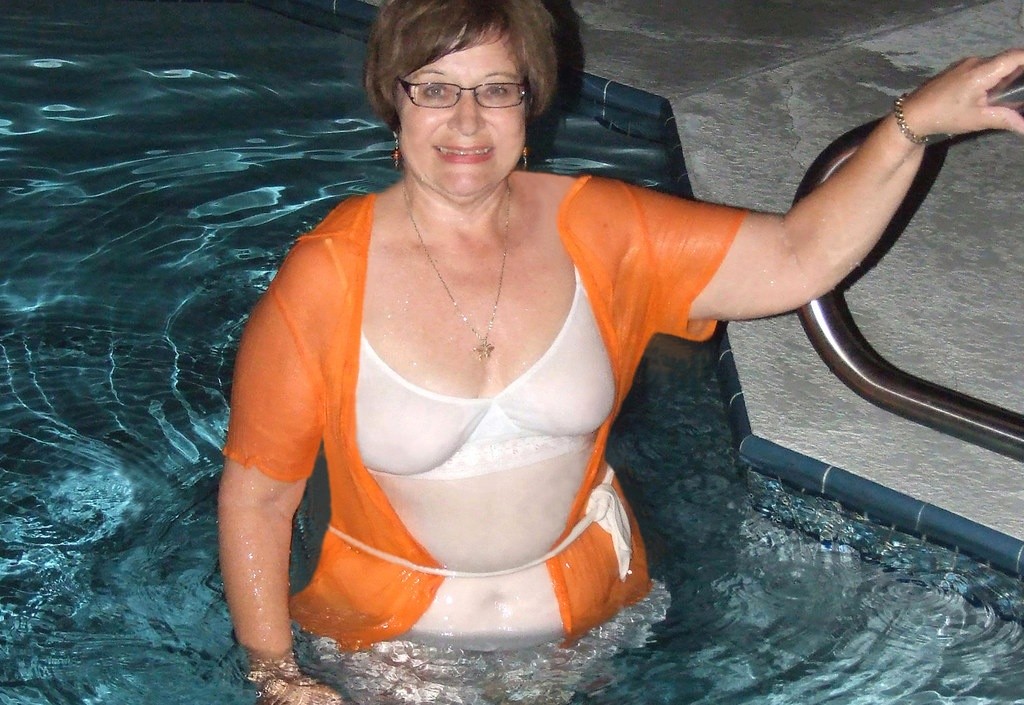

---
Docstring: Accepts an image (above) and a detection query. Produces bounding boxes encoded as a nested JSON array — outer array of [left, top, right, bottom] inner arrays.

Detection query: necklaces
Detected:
[[400, 177, 514, 358]]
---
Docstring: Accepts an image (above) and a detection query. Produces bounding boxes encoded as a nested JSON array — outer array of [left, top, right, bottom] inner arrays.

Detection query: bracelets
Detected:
[[894, 92, 931, 143]]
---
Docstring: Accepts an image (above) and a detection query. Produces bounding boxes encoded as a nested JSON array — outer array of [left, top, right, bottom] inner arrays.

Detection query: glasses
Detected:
[[397, 77, 529, 108]]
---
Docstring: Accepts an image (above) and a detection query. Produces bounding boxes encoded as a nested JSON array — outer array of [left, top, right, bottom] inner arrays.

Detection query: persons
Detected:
[[217, 1, 1024, 705]]
